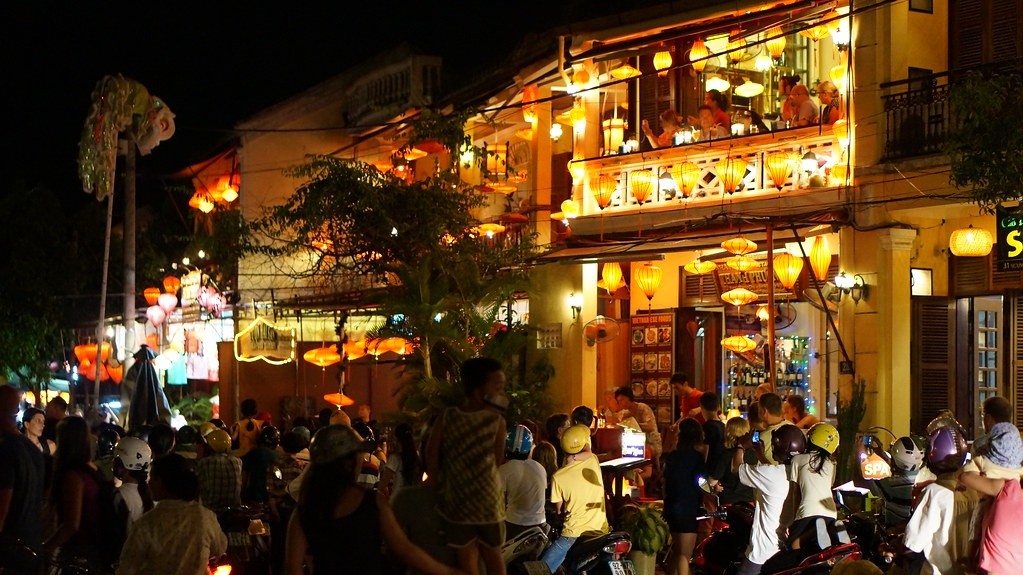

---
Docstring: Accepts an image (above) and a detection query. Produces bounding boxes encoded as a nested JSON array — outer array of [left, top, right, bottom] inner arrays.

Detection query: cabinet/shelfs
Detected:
[[726, 359, 802, 414]]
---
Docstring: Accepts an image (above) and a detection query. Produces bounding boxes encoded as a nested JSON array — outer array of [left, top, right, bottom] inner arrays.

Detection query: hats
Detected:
[[973, 422, 1023, 476]]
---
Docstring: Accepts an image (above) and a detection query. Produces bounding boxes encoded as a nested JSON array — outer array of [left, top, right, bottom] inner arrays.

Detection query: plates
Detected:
[[631, 327, 672, 421]]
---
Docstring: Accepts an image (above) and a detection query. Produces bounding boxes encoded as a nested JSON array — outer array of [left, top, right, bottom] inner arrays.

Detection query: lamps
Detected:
[[484, 125, 509, 175], [548, 122, 562, 142], [834, 270, 869, 306], [659, 167, 673, 191], [949, 223, 994, 257], [801, 147, 817, 172]]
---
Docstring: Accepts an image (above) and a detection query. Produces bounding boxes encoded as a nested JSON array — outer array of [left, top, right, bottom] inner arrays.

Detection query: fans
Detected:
[[760, 300, 797, 331], [582, 315, 621, 346], [726, 41, 762, 68], [684, 47, 720, 83], [803, 281, 839, 314]]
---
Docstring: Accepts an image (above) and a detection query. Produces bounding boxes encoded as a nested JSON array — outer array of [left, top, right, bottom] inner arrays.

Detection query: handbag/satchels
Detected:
[[231, 436, 240, 451]]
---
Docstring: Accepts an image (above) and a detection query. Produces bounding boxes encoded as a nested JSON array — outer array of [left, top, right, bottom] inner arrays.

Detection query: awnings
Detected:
[[169, 147, 241, 200], [568, 0, 850, 64]]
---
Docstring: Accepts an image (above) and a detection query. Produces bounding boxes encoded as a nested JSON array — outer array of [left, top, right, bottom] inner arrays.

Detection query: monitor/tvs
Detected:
[[621, 432, 645, 458]]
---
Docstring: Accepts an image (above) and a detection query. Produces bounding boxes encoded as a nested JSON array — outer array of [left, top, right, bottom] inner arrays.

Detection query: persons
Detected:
[[642, 75, 842, 150], [604, 373, 1023, 575], [0, 356, 609, 575]]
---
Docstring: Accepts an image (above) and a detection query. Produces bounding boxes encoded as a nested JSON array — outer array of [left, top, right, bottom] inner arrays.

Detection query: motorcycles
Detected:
[[837, 489, 908, 575], [690, 476, 863, 575], [500, 526, 549, 575], [211, 501, 270, 564], [552, 530, 637, 575]]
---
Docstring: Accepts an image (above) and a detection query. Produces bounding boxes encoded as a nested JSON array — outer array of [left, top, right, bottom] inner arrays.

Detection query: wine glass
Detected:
[[692, 130, 700, 142]]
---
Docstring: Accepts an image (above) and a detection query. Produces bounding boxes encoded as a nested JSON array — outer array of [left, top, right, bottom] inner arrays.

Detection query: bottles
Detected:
[[726, 345, 810, 411]]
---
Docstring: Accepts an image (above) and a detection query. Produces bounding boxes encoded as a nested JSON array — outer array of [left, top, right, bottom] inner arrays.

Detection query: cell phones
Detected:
[[752, 430, 760, 445], [859, 436, 873, 445]]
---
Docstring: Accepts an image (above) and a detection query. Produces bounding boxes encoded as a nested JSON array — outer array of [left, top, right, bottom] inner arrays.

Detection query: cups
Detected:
[[787, 119, 793, 128], [710, 127, 718, 140], [777, 121, 785, 129], [598, 419, 605, 428], [750, 124, 759, 134]]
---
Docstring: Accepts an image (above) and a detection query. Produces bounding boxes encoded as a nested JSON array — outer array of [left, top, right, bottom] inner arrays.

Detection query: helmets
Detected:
[[98, 429, 120, 454], [113, 436, 152, 470], [771, 424, 807, 458], [924, 426, 968, 475], [891, 437, 923, 472], [178, 425, 196, 444], [560, 423, 591, 454], [292, 426, 310, 442], [200, 422, 217, 438], [806, 421, 840, 454], [259, 425, 280, 448], [504, 424, 533, 454], [206, 430, 233, 455], [308, 423, 364, 466]]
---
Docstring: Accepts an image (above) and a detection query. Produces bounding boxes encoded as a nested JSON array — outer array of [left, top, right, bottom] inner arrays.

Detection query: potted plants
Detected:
[[623, 502, 670, 575]]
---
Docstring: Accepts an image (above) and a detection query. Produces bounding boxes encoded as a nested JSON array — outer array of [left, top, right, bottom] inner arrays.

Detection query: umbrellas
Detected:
[[119, 344, 173, 438]]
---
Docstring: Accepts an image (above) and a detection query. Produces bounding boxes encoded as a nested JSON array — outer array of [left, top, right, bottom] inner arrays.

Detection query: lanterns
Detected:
[[589, 116, 853, 242], [303, 336, 416, 410], [188, 174, 240, 214], [366, 69, 590, 240], [949, 223, 993, 257], [598, 234, 831, 382], [144, 277, 226, 357], [74, 337, 123, 387], [610, 11, 848, 113], [306, 219, 352, 267]]
